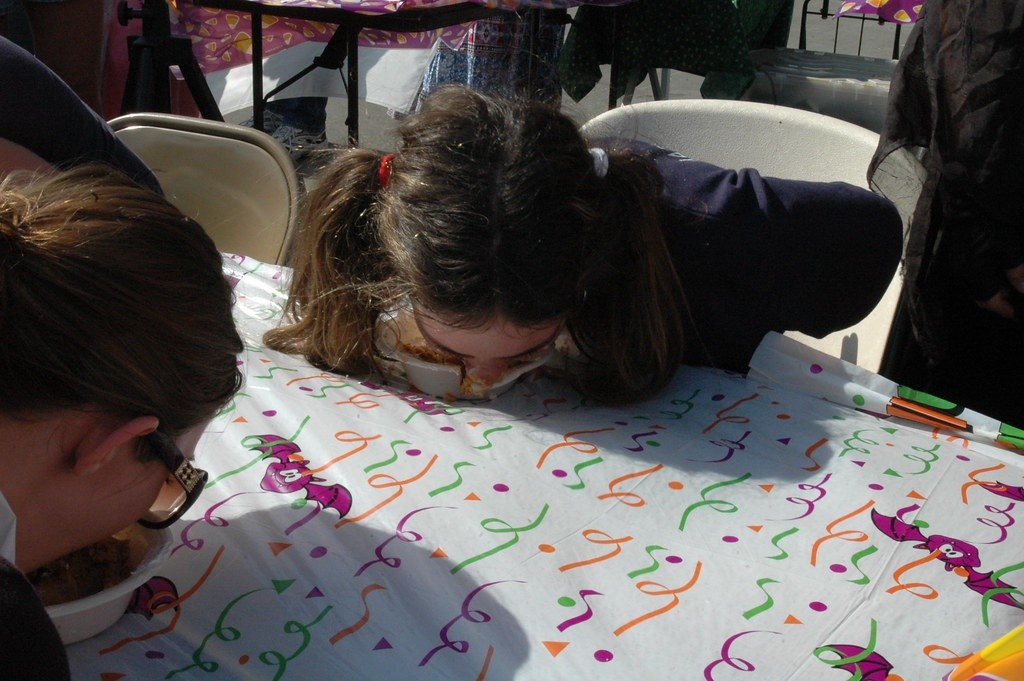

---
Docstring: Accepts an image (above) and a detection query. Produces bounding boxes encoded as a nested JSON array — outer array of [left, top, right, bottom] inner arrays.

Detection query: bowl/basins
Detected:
[[26, 513, 175, 642], [373, 312, 556, 400]]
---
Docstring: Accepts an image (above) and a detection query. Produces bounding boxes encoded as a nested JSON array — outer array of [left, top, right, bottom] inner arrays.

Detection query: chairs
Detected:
[[106, 114, 299, 265], [579, 99, 927, 373]]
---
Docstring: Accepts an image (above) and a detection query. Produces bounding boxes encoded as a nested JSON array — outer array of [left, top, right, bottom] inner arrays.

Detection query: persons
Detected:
[[865, 0, 1024, 432], [0, 35, 163, 194], [261, 82, 905, 409], [0, 166, 246, 681]]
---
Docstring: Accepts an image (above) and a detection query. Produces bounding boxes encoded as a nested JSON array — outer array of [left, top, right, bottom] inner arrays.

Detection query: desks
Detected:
[[101, 0, 520, 147]]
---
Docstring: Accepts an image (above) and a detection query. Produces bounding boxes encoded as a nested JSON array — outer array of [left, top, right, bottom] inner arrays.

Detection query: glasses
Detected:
[[138, 431, 208, 529]]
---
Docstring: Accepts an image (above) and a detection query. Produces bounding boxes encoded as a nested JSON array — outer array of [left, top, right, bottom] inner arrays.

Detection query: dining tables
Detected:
[[65, 254, 1024, 681]]
